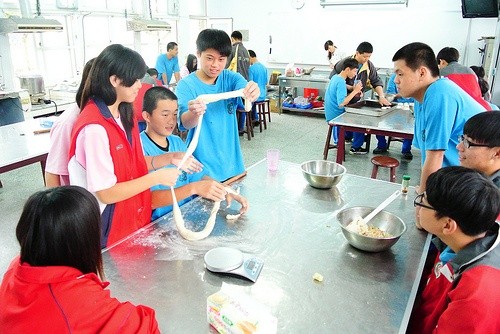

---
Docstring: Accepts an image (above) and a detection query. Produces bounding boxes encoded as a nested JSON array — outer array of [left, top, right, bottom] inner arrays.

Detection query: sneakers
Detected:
[[349, 146, 368, 155]]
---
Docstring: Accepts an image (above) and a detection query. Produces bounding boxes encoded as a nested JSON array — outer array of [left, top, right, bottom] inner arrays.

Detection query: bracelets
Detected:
[[151, 156, 157, 171], [379, 96, 385, 98]]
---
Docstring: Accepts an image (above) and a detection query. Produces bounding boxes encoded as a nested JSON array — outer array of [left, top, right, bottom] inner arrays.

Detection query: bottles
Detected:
[[282, 87, 293, 98], [401, 175, 411, 195]]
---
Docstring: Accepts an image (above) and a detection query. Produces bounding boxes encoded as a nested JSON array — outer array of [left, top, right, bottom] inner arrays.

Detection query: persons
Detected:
[[44, 57, 96, 186], [66, 43, 203, 249], [436, 47, 500, 113], [133, 41, 199, 131], [139, 86, 249, 224], [456, 109, 500, 225], [224, 31, 268, 137], [324, 58, 369, 155], [0, 185, 160, 334], [324, 40, 342, 70], [329, 42, 391, 145], [392, 41, 488, 194], [372, 69, 416, 162], [176, 29, 260, 198], [405, 166, 500, 334]]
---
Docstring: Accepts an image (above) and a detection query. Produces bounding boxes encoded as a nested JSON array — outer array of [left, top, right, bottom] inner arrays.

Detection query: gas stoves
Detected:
[[30, 95, 45, 104]]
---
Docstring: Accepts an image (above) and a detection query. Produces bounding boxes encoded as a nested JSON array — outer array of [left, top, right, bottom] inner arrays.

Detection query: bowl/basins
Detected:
[[310, 100, 323, 107], [300, 160, 347, 189], [336, 206, 407, 253]]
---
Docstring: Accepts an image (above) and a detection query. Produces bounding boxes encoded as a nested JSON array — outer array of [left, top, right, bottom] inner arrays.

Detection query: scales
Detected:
[[204, 246, 264, 282]]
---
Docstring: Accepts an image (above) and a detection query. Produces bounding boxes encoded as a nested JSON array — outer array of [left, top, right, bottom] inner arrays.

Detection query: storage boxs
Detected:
[[282, 88, 323, 109]]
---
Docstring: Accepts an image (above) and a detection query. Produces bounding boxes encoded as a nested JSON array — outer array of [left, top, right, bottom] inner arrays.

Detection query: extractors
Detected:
[[0, 0, 63, 33], [129, 0, 171, 30]]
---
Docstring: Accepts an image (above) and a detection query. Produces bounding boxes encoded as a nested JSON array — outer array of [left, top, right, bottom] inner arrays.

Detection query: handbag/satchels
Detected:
[[228, 44, 239, 73]]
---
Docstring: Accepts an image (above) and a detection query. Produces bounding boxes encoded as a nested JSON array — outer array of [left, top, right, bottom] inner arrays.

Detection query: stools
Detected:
[[324, 125, 337, 160], [237, 99, 271, 140], [371, 156, 399, 182]]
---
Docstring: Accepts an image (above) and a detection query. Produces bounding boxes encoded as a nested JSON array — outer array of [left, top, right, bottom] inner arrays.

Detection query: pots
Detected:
[[20, 74, 44, 94]]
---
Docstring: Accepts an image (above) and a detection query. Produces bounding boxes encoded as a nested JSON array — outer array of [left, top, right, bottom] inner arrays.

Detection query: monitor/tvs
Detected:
[[461, 0, 500, 18]]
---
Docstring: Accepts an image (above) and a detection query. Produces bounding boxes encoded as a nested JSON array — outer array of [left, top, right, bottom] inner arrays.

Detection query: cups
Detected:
[[396, 103, 414, 110], [267, 148, 280, 174]]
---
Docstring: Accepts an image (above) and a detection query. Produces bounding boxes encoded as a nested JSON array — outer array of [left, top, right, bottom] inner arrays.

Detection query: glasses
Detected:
[[457, 134, 493, 149], [413, 194, 437, 212]]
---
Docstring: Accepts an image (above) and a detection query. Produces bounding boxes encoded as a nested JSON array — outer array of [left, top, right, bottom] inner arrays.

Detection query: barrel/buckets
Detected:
[[304, 88, 319, 102]]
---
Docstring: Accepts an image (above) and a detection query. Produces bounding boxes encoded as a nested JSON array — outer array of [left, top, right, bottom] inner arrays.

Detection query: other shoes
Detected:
[[401, 151, 413, 160], [373, 147, 388, 153], [252, 121, 259, 127], [344, 140, 353, 145]]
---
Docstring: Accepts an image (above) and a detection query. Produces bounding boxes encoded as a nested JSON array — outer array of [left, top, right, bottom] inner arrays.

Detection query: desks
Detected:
[[0, 115, 58, 188], [328, 109, 414, 165], [101, 159, 433, 334]]
